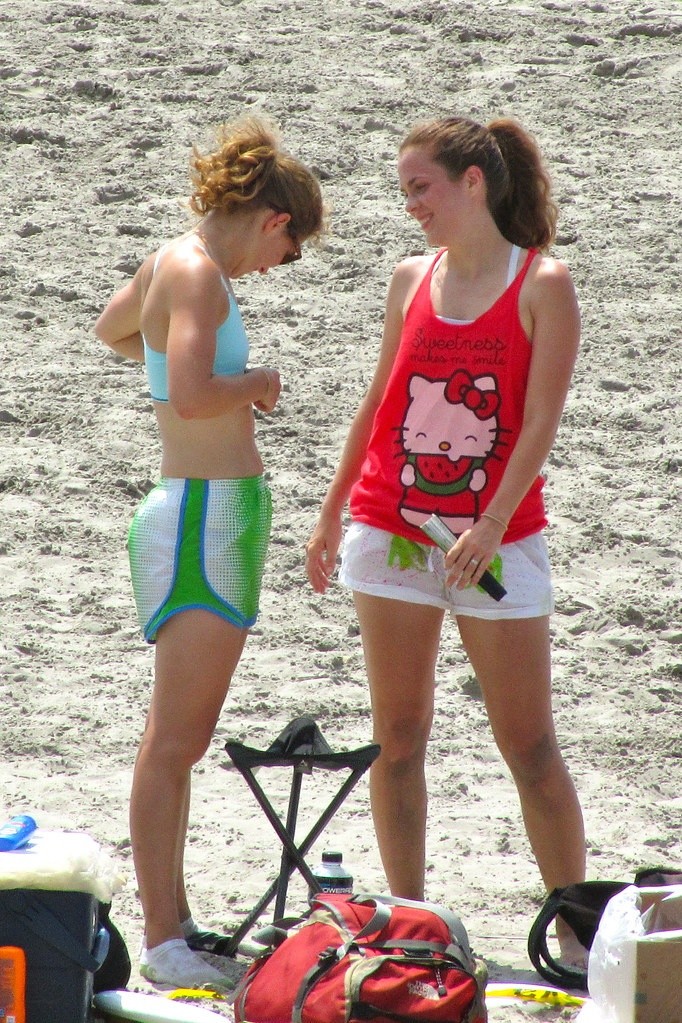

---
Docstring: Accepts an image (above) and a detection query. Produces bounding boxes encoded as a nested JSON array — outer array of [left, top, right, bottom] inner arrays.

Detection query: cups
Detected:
[[312, 851, 353, 893]]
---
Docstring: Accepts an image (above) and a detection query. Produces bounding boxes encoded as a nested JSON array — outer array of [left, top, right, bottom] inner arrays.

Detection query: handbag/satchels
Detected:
[[234, 894, 487, 1023]]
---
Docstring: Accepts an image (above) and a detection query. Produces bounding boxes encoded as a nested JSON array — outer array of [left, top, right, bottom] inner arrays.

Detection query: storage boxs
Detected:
[[0, 823, 132, 1023], [624, 885, 682, 1023]]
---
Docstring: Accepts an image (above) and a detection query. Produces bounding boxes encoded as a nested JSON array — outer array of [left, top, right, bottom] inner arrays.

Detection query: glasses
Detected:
[[266, 201, 302, 268]]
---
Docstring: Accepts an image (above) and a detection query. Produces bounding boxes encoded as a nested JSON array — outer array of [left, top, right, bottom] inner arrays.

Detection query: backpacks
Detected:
[[526, 867, 682, 991]]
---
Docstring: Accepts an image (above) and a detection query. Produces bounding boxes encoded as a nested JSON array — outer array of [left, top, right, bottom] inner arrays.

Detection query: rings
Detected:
[[471, 560, 478, 565]]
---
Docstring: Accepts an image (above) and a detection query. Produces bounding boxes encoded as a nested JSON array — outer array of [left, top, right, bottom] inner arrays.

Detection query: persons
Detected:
[[93, 122, 324, 992], [306, 116, 589, 965]]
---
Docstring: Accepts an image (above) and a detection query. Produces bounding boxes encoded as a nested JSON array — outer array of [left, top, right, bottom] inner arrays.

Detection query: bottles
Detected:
[[0, 815, 37, 852]]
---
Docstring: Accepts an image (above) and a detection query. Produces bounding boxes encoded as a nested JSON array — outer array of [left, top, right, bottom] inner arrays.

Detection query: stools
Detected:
[[215, 717, 382, 955]]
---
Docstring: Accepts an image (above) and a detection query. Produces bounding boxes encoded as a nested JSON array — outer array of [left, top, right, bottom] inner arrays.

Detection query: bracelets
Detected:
[[481, 513, 509, 530], [264, 370, 271, 393]]
